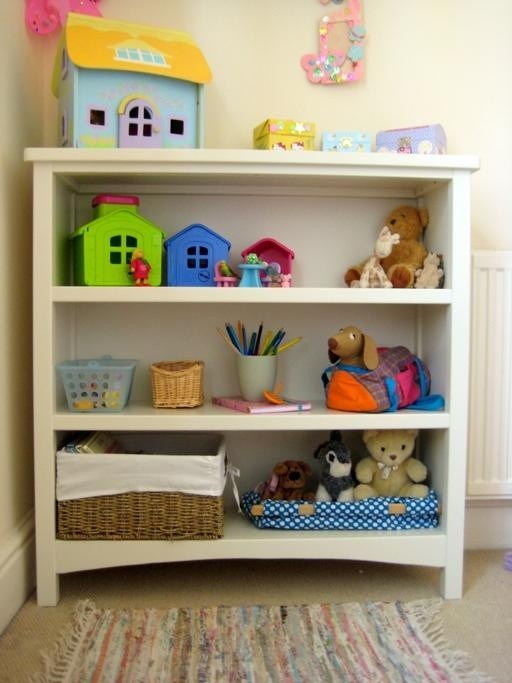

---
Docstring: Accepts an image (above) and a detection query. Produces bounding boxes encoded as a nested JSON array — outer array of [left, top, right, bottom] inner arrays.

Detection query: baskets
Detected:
[[53, 431, 229, 542], [149, 358, 205, 409]]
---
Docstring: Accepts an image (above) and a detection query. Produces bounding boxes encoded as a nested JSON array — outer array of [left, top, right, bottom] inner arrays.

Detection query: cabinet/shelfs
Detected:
[[22, 148, 480, 608]]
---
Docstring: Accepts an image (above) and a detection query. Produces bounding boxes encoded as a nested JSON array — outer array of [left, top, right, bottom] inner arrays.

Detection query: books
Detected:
[[65, 430, 123, 453]]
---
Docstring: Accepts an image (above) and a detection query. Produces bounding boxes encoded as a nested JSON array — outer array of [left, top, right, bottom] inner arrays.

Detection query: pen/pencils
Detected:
[[216, 319, 302, 356]]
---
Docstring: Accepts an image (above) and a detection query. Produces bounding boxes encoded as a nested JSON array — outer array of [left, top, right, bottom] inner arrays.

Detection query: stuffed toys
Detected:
[[328, 327, 380, 370], [252, 429, 429, 503], [344, 205, 445, 289]]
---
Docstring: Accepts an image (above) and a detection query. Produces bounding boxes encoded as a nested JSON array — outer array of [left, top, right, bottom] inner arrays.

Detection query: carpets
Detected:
[[25, 599, 493, 683]]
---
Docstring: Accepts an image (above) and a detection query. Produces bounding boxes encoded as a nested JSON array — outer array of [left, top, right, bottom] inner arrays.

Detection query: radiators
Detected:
[[469, 251, 512, 499]]
